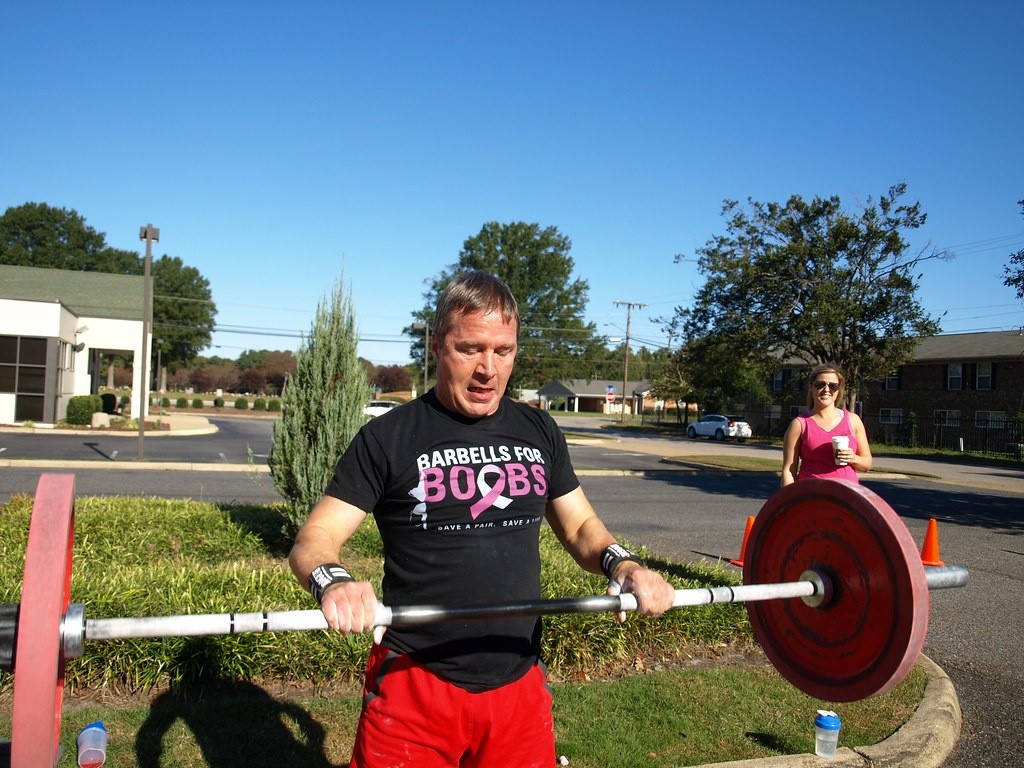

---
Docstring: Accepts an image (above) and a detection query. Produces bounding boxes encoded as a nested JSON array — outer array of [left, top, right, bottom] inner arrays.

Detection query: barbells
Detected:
[[0, 465, 967, 768]]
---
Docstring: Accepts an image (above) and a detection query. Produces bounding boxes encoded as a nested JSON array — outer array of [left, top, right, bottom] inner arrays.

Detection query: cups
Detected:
[[832, 436, 849, 465]]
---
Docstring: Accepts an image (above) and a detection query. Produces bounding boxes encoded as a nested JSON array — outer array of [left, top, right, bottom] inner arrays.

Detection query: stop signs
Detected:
[[607, 391, 614, 402]]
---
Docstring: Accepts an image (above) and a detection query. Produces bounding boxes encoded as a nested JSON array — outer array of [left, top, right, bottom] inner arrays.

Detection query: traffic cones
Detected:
[[730, 515, 757, 570], [919, 518, 946, 569]]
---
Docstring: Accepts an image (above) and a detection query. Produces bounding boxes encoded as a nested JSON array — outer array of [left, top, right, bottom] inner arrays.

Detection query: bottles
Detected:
[[815, 710, 841, 759], [77, 721, 108, 768]]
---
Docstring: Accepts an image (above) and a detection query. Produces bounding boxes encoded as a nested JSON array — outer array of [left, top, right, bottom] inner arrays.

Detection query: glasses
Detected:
[[813, 380, 840, 392]]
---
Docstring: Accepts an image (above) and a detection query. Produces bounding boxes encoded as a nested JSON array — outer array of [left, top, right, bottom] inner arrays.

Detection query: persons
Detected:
[[289, 268, 675, 768], [780, 363, 872, 488]]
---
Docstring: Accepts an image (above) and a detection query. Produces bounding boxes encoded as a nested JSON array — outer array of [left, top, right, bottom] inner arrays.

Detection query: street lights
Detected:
[[411, 322, 430, 394], [138, 223, 161, 457], [604, 321, 631, 426]]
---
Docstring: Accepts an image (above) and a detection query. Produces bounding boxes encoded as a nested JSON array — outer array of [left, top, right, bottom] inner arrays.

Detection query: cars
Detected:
[[361, 400, 403, 422], [685, 415, 752, 443]]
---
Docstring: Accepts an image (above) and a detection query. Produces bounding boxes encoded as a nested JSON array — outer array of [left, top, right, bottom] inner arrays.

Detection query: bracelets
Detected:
[[599, 543, 643, 579], [308, 563, 355, 604]]
